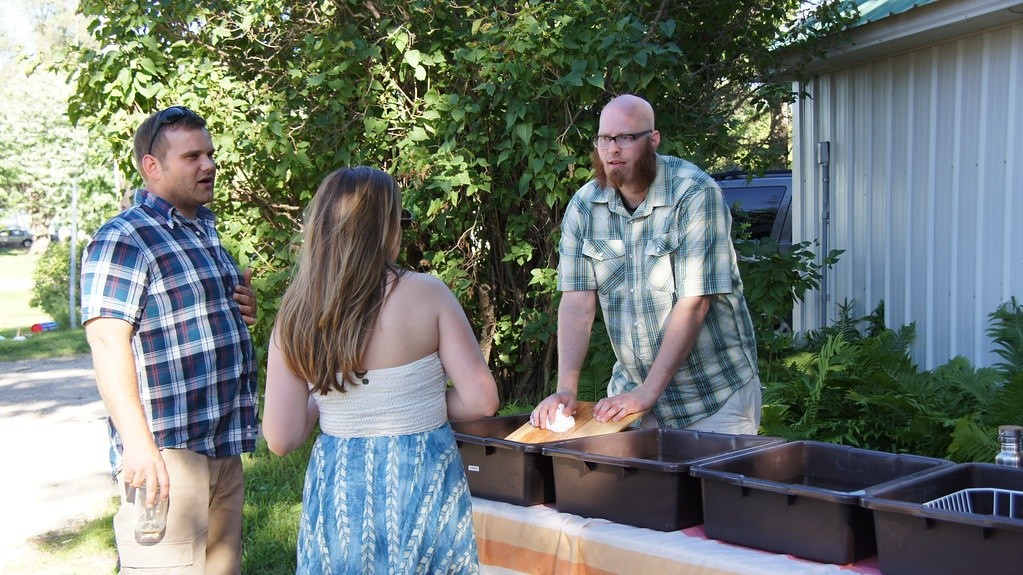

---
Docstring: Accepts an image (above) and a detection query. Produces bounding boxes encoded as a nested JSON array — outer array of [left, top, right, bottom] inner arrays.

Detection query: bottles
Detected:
[[135, 480, 168, 542]]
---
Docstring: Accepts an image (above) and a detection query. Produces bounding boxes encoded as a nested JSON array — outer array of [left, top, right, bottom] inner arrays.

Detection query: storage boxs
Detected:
[[449, 412, 1023, 575]]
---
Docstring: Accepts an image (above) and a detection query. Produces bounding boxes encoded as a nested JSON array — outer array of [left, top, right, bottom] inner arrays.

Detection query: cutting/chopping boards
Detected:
[[504, 402, 651, 443]]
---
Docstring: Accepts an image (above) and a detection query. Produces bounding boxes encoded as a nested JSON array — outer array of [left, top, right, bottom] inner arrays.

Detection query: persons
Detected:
[[79, 106, 258, 575], [530, 94, 762, 436], [262, 165, 499, 575]]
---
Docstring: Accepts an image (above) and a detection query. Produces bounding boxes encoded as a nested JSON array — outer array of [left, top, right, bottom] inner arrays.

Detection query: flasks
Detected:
[[995, 425, 1023, 469]]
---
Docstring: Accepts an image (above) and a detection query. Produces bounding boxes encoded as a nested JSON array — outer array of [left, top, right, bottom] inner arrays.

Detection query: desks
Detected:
[[471, 494, 881, 574]]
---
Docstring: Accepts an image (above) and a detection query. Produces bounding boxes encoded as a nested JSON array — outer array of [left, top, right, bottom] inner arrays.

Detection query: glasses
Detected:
[[592, 129, 653, 150], [146, 106, 196, 155]]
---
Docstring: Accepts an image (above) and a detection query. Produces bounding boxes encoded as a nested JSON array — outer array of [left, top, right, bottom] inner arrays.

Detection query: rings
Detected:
[[618, 403, 623, 409]]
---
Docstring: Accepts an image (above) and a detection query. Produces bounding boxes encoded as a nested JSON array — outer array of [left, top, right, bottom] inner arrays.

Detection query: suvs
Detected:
[[708, 169, 795, 261]]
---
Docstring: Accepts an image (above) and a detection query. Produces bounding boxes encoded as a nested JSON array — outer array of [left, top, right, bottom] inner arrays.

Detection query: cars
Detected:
[[0, 229, 34, 250]]
